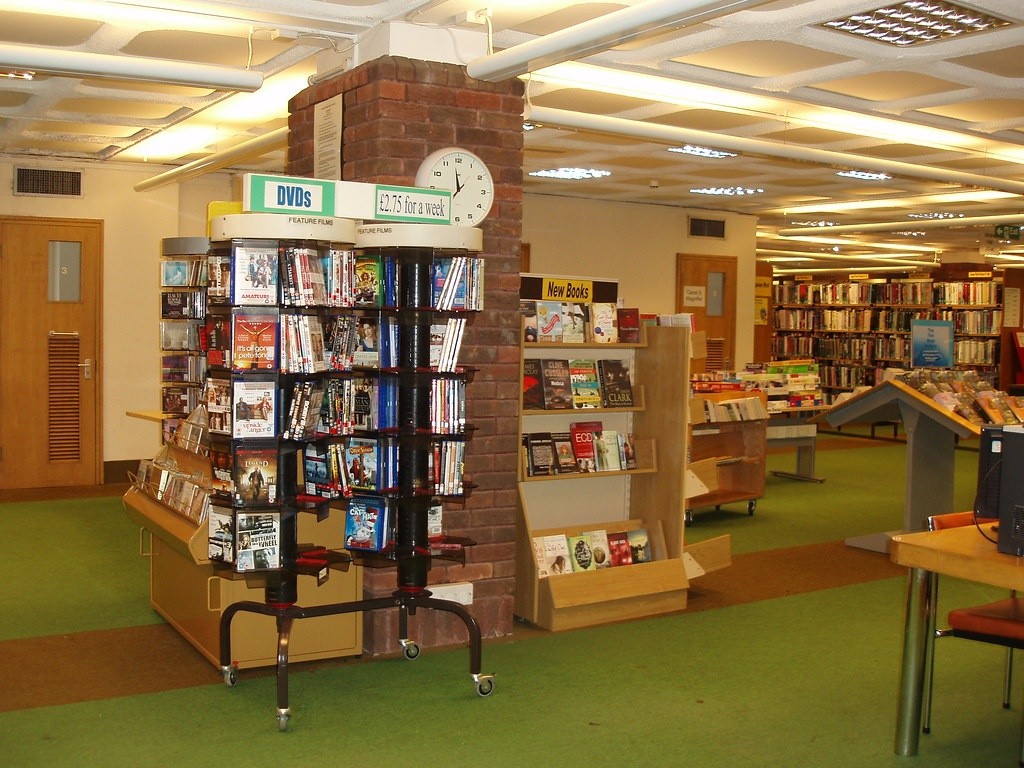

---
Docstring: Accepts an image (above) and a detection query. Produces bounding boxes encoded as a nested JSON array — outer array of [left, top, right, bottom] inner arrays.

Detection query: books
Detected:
[[234, 511, 281, 575], [207, 503, 234, 568], [532, 528, 652, 579], [768, 280, 1024, 430], [519, 300, 823, 477], [135, 245, 485, 553]]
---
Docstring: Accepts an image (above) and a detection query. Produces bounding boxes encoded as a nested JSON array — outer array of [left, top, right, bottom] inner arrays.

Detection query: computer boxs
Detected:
[[998, 426, 1024, 556]]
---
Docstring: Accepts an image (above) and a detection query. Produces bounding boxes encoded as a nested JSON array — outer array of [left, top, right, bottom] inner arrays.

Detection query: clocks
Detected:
[[414, 147, 495, 228]]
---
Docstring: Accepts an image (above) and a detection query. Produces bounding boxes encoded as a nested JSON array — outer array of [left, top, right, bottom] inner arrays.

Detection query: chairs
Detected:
[[922, 512, 1024, 768]]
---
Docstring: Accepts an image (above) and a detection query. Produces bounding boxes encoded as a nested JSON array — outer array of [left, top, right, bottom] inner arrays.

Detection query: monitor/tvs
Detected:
[[973, 424, 1003, 518]]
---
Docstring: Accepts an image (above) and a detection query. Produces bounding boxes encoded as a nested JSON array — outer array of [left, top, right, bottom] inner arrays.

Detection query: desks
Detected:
[[768, 404, 834, 483], [889, 522, 1024, 768]]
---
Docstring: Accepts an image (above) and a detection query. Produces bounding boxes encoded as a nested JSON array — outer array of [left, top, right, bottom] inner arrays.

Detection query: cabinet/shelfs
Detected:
[[123, 214, 485, 672], [685, 389, 769, 513], [516, 300, 693, 633], [772, 301, 1003, 391]]
